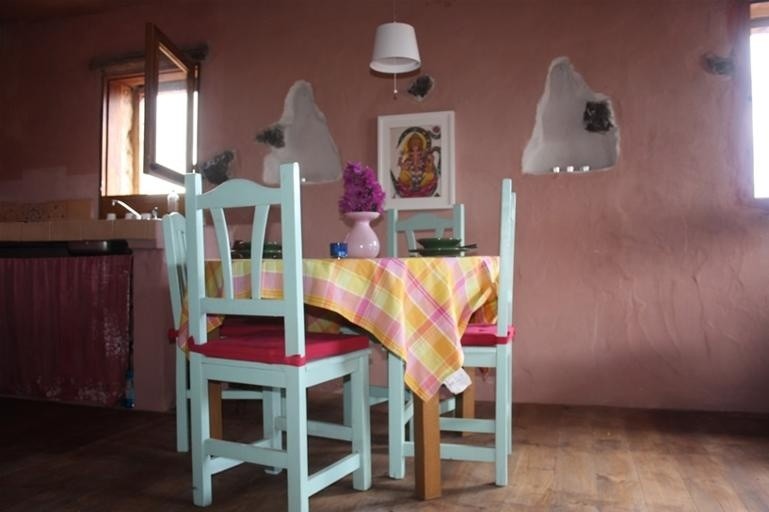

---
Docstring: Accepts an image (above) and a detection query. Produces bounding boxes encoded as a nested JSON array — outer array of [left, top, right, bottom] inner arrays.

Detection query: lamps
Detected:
[[368, 1, 422, 96]]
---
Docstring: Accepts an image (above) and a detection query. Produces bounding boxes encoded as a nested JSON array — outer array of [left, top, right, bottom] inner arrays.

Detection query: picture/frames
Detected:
[[376, 109, 458, 212]]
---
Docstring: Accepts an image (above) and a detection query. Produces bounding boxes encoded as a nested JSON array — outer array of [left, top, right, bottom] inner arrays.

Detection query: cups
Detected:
[[329, 243, 347, 259]]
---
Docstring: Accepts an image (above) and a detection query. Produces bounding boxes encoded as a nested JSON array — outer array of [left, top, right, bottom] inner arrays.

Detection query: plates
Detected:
[[229, 241, 281, 259], [410, 239, 470, 257]]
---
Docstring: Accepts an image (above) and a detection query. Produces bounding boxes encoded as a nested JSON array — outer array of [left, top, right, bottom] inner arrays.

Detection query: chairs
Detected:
[[160, 212, 286, 452], [183, 162, 374, 511], [338, 203, 464, 428], [388, 178, 517, 487]]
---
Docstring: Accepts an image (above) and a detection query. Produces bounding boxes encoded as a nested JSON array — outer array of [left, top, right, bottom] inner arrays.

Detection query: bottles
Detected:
[[167, 189, 179, 212]]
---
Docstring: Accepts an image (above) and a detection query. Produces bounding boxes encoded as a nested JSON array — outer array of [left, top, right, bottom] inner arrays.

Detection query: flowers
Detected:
[[334, 162, 387, 212]]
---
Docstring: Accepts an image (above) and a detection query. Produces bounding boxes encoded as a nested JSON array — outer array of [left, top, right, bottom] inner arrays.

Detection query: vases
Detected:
[[343, 208, 382, 258]]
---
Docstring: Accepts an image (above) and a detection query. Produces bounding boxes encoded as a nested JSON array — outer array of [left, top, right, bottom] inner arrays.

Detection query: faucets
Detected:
[[110, 197, 143, 220]]
